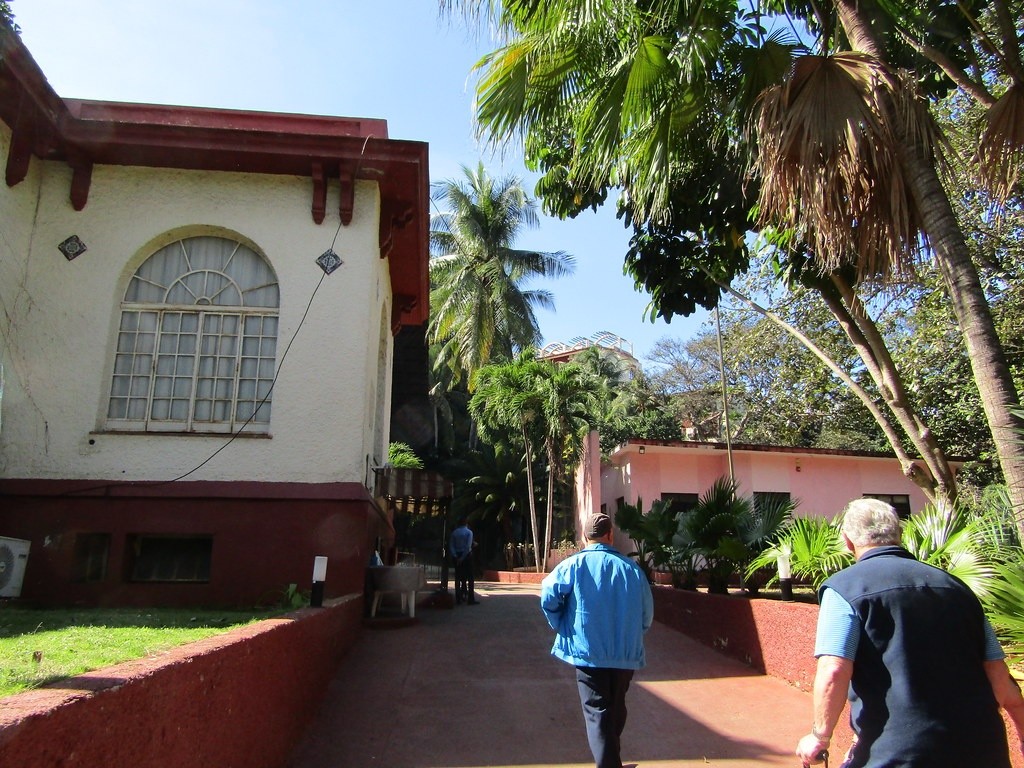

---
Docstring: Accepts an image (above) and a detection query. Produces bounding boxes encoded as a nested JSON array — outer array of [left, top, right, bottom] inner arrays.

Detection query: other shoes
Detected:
[[467, 600, 479, 605], [457, 599, 463, 604]]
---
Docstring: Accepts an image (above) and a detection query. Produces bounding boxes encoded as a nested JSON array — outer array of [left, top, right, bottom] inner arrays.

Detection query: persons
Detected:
[[796, 499, 1024, 768], [448, 517, 480, 605], [541, 512, 654, 768]]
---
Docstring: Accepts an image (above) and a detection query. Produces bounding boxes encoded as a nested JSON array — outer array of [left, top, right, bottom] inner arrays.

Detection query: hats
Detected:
[[585, 512, 613, 537]]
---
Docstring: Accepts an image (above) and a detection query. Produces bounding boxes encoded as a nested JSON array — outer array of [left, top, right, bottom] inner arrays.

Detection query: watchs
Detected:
[[812, 721, 831, 741]]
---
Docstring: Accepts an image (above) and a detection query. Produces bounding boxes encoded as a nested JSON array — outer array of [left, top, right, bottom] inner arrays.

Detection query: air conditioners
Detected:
[[0, 536, 31, 598]]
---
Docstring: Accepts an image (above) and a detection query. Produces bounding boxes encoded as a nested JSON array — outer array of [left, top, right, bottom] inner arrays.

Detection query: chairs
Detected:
[[368, 566, 426, 618]]
